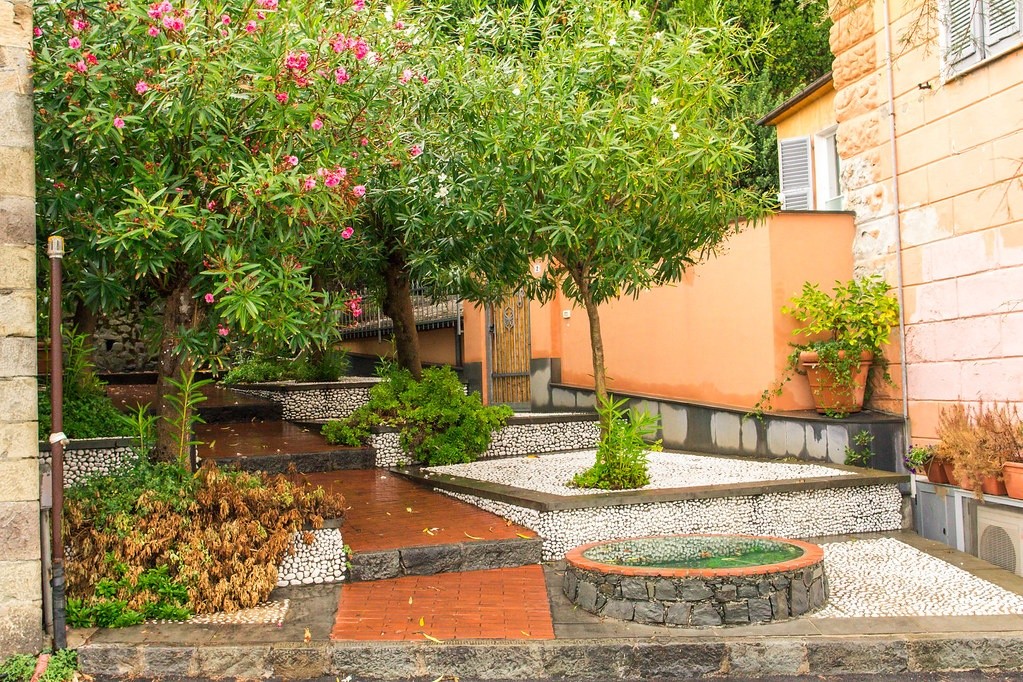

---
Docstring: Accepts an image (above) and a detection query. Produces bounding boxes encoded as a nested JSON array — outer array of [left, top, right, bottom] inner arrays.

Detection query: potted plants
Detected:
[[741, 273, 901, 430], [920, 396, 1023, 502]]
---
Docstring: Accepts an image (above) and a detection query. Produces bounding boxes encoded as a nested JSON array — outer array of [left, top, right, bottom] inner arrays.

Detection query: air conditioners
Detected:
[[976, 504, 1023, 578]]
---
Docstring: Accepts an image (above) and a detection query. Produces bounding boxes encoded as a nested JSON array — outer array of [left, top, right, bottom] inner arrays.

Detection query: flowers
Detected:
[[902, 443, 933, 475]]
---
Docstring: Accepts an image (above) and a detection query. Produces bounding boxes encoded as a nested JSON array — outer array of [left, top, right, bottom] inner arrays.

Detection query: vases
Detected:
[[922, 458, 950, 485]]
